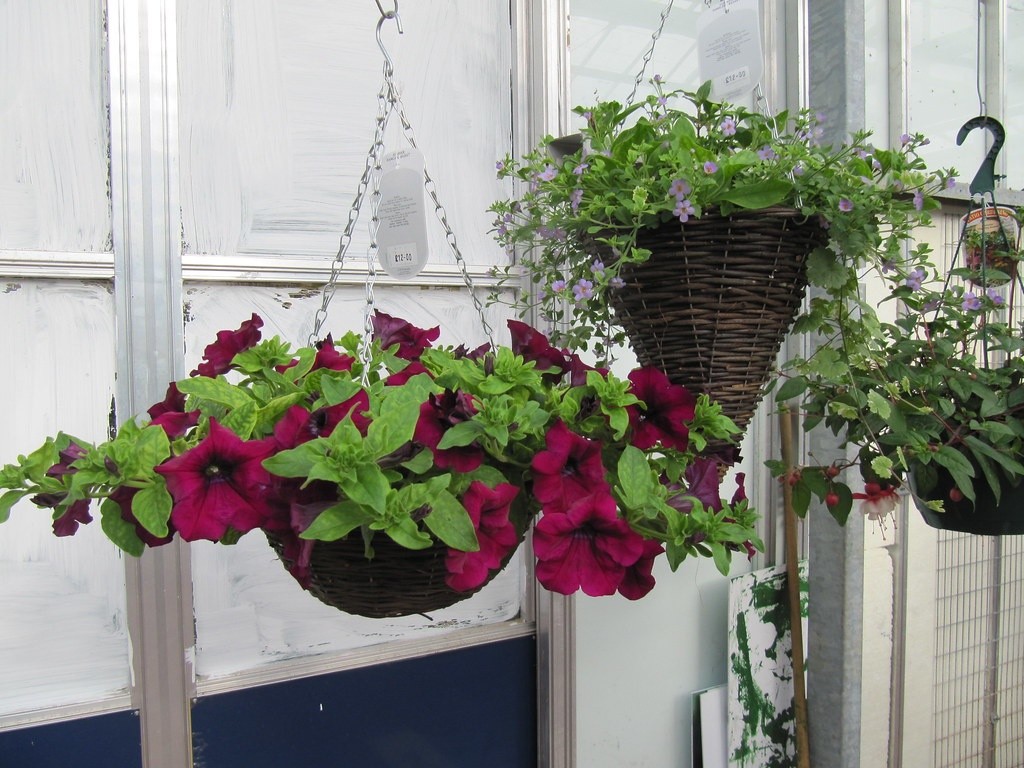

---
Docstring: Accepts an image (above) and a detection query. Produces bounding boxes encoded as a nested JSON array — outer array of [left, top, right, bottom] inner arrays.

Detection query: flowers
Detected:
[[485, 75, 1024, 514], [0, 309, 768, 601], [766, 289, 1024, 540]]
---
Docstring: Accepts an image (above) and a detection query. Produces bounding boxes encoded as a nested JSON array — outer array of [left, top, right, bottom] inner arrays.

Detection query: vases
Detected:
[[899, 427, 1024, 535]]
